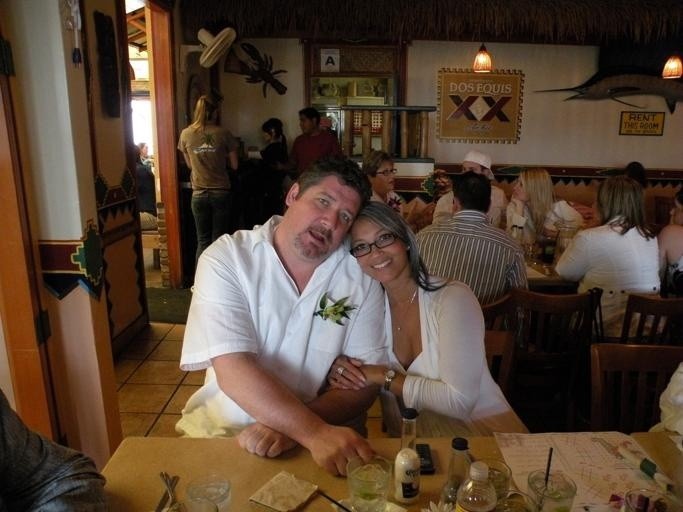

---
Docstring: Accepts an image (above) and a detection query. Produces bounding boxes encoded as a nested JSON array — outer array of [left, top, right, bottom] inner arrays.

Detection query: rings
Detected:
[[335, 366, 345, 375]]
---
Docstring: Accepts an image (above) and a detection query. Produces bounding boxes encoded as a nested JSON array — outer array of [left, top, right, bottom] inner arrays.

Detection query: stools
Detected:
[[140, 229, 161, 271]]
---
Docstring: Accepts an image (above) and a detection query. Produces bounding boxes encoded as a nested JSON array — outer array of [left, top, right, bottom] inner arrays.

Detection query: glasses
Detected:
[[349, 232, 398, 257], [378, 169, 397, 176]]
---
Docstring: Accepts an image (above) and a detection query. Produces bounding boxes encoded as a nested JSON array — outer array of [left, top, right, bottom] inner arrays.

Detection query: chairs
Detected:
[[473, 257, 682, 435]]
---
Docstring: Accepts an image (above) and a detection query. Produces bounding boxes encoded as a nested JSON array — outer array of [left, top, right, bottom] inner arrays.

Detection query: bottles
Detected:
[[391, 408, 419, 450], [438, 437, 468, 511], [394, 452, 420, 504], [453, 460, 497, 512]]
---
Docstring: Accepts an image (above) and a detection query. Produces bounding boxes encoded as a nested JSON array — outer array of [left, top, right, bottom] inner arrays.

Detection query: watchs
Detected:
[[383, 367, 398, 391]]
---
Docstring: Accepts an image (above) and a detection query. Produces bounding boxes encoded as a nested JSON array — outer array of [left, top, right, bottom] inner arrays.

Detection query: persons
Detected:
[[171, 154, 390, 479], [132, 93, 342, 266], [324, 201, 531, 438], [358, 149, 683, 438], [0, 384, 105, 512]]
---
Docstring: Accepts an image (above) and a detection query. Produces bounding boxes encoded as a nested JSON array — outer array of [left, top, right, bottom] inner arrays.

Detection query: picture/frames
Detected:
[[315, 107, 341, 142]]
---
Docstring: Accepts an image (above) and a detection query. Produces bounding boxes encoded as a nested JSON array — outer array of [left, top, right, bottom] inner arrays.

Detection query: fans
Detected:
[[178, 26, 236, 76]]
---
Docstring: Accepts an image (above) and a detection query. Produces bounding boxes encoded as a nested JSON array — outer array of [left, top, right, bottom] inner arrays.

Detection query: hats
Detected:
[[463, 151, 494, 179]]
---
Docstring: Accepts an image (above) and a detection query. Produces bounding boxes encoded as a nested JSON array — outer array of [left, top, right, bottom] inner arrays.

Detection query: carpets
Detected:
[[145, 285, 193, 327]]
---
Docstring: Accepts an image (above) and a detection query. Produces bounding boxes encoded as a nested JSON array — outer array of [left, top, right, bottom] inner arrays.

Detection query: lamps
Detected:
[[661, 0, 682, 80], [472, 0, 492, 74]]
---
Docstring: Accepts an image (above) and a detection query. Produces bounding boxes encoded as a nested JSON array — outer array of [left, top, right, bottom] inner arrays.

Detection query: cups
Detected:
[[498, 490, 536, 511], [345, 458, 392, 511], [186, 474, 231, 512], [478, 458, 511, 498], [623, 487, 670, 512], [168, 497, 217, 512], [527, 468, 575, 512]]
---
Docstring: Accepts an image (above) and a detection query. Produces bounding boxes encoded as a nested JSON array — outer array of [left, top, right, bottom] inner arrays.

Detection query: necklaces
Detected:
[[384, 287, 420, 333]]
